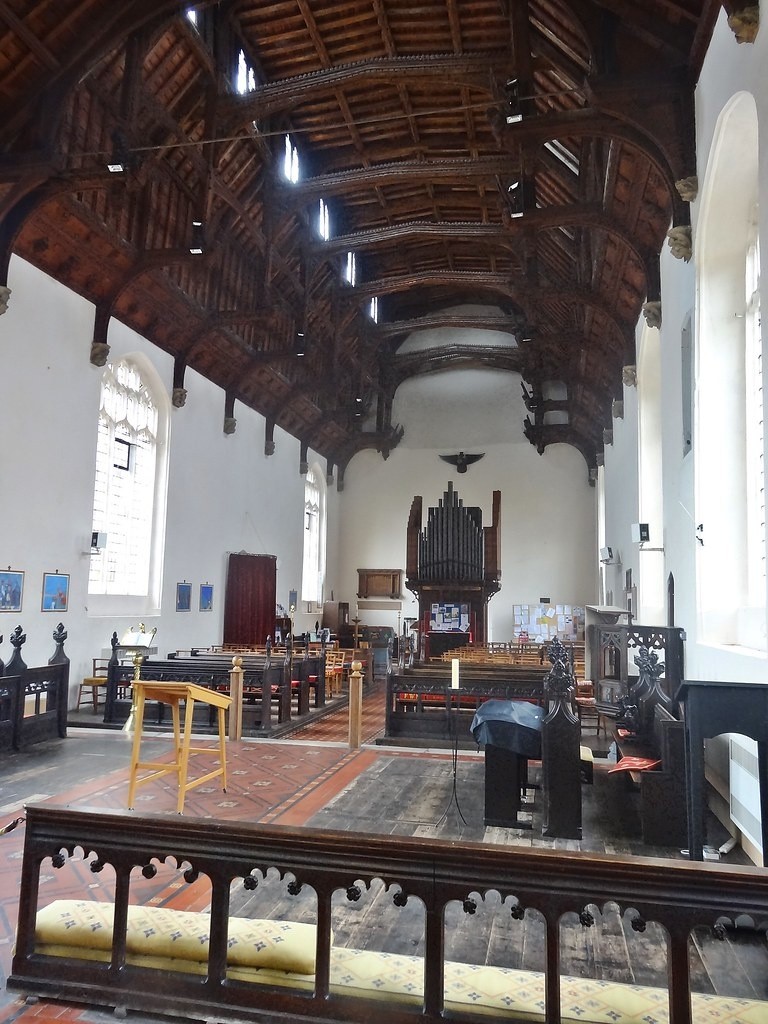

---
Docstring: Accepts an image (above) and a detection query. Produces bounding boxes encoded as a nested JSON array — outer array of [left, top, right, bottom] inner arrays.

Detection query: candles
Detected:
[[355, 605, 359, 616]]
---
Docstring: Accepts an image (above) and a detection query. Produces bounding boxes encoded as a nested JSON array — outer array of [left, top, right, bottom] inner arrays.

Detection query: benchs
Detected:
[[611, 702, 707, 848], [376, 659, 585, 751], [102, 649, 327, 736]]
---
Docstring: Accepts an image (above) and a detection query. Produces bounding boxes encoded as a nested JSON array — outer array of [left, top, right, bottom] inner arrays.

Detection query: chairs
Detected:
[[177, 642, 585, 699], [77, 659, 110, 715], [574, 679, 608, 741]]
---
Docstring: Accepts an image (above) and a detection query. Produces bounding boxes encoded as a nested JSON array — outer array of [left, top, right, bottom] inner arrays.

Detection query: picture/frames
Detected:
[[0, 570, 25, 612], [41, 572, 70, 612], [176, 583, 192, 613], [199, 584, 214, 612], [626, 568, 632, 590]]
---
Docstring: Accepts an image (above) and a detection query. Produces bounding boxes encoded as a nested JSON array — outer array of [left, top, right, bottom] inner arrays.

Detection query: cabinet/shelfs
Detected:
[[322, 600, 349, 648]]
